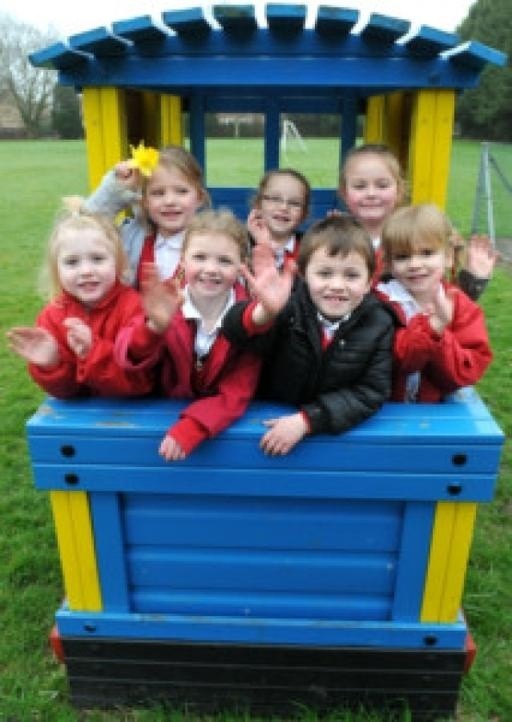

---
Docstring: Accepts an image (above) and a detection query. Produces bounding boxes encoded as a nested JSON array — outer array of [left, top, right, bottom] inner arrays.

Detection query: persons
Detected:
[[3, 196, 154, 400], [371, 207, 493, 403], [246, 168, 310, 271], [326, 141, 500, 302], [81, 144, 206, 290], [215, 217, 394, 458], [113, 210, 262, 462]]
